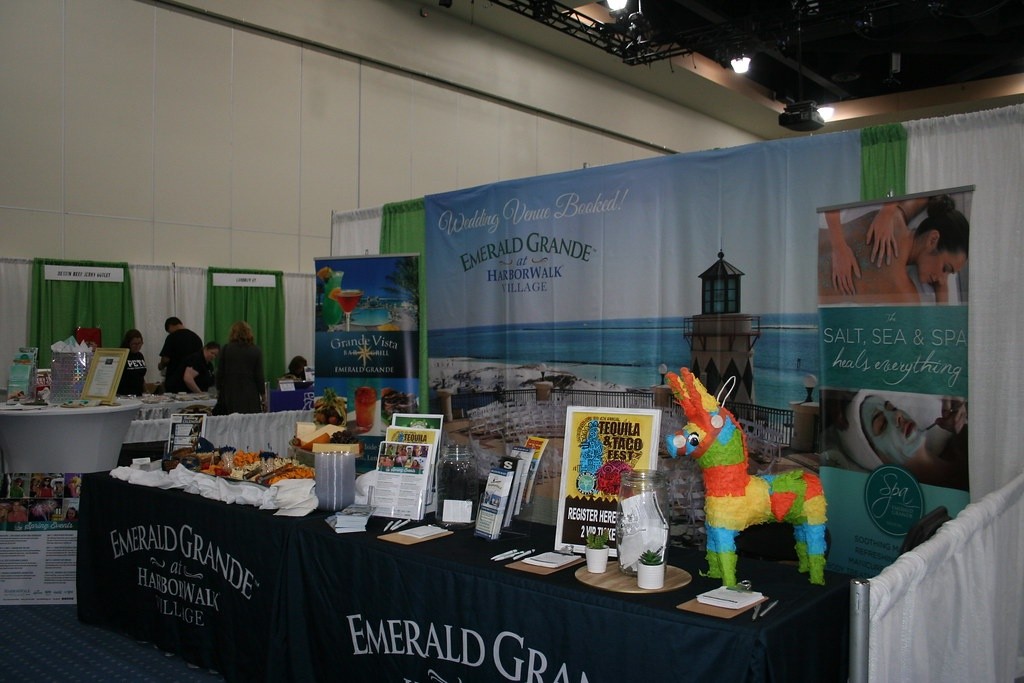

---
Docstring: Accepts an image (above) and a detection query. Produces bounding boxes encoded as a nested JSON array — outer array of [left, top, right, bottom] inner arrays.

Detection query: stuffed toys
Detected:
[[662, 367, 828, 586]]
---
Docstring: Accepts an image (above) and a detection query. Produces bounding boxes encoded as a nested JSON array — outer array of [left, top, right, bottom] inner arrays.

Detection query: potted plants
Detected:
[[638, 550, 665, 589], [585, 528, 609, 574]]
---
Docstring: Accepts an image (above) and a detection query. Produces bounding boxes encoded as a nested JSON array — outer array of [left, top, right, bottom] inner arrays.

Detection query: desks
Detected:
[[75, 470, 847, 683], [0, 401, 145, 474], [116, 392, 218, 421]]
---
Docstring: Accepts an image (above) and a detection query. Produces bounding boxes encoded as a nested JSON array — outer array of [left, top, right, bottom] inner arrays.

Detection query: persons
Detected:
[[827, 389, 969, 491], [158, 317, 203, 394], [282, 355, 308, 380], [212, 321, 265, 415], [164, 341, 221, 394], [819, 194, 970, 305], [117, 328, 147, 396]]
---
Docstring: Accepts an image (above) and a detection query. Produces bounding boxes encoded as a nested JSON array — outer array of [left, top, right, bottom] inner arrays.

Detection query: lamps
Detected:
[[539, 363, 546, 381], [803, 374, 817, 402], [439, 369, 448, 389], [658, 364, 667, 385]]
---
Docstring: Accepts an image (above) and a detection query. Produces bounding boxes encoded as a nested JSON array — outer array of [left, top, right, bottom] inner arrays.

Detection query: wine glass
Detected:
[[334, 290, 366, 331]]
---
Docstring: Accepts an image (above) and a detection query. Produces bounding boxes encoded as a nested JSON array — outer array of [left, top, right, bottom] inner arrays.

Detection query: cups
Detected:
[[355, 386, 376, 428]]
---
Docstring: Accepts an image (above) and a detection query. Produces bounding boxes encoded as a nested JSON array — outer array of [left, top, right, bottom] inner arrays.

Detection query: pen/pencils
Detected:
[[752, 604, 761, 621], [384, 518, 410, 532], [759, 600, 778, 617], [491, 549, 535, 561]]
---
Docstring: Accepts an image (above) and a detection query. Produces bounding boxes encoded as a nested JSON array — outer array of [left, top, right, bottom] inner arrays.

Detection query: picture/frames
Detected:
[[80, 347, 130, 405]]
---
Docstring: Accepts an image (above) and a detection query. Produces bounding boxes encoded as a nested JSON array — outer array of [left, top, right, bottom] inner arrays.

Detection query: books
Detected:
[[324, 504, 377, 534]]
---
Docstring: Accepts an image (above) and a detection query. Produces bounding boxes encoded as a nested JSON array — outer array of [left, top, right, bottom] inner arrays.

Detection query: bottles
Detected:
[[615, 468, 668, 576], [435, 444, 478, 527]]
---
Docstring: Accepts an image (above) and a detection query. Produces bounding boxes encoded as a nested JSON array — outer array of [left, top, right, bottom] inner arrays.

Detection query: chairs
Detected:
[[466, 400, 784, 462]]
